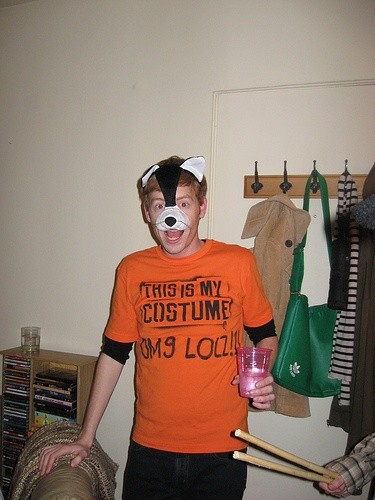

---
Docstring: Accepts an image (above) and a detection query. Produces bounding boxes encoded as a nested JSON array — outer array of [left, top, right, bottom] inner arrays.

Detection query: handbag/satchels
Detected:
[[271, 170, 342, 398]]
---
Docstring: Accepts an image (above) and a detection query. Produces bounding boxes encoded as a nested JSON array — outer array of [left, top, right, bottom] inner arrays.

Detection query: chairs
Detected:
[[10, 421, 120, 500]]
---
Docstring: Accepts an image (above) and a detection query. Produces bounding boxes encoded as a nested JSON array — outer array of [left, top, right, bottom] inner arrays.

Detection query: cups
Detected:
[[236, 347, 272, 399], [20, 327, 41, 354]]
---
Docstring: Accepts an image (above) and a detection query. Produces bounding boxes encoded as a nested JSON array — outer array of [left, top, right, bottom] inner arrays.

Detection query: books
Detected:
[[1, 355, 77, 485]]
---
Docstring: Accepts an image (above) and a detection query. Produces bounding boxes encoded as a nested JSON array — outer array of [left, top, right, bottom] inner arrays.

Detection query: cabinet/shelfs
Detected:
[[0, 345, 99, 500]]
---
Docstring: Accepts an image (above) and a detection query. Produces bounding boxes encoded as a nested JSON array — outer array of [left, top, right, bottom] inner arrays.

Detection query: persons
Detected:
[[319, 432, 375, 499], [37, 154, 278, 499]]
